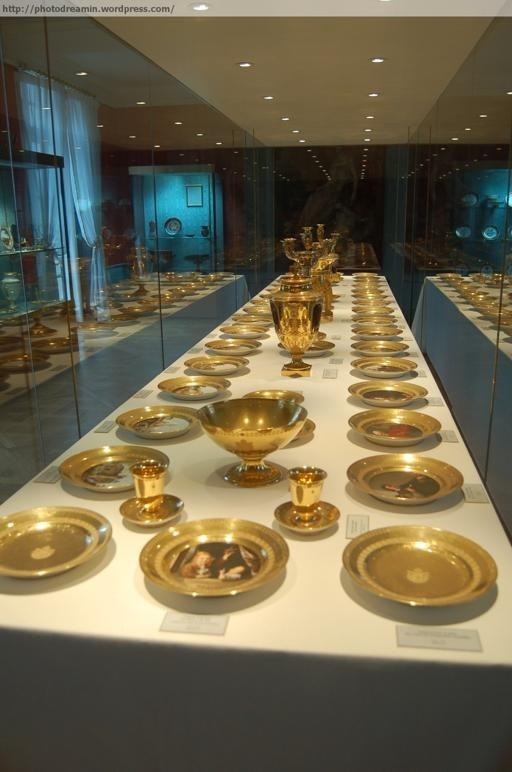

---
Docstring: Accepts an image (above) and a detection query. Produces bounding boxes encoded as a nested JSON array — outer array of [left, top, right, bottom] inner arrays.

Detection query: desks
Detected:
[[1, 274, 512, 772], [411, 275, 512, 537], [0, 271, 247, 503]]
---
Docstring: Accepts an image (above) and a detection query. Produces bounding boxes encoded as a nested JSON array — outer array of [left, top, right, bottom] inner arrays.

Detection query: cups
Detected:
[[287, 465, 327, 523], [129, 459, 167, 515]]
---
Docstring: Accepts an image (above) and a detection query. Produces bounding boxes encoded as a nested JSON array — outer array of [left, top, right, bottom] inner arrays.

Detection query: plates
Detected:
[[271, 496, 343, 537], [119, 496, 184, 527], [435, 264, 512, 338], [341, 525, 499, 607], [0, 223, 20, 251], [3, 266, 502, 405], [116, 407, 199, 440], [163, 218, 183, 237], [0, 506, 108, 580], [141, 515, 291, 602], [347, 407, 443, 449], [345, 455, 465, 508], [211, 238, 485, 276], [59, 446, 170, 494], [454, 191, 512, 238]]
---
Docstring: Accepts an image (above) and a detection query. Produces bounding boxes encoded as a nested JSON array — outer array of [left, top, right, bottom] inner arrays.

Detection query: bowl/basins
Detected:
[[197, 398, 305, 486]]
